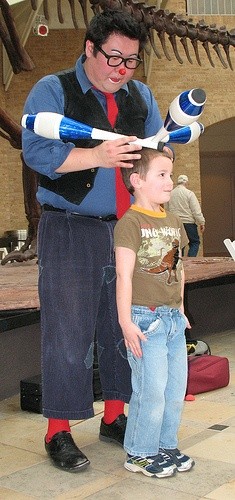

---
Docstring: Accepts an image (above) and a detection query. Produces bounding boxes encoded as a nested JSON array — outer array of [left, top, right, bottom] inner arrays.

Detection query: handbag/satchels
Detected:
[[186, 341, 231, 395]]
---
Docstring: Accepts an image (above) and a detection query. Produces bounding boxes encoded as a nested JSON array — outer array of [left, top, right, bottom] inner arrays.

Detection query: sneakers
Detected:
[[124, 452, 178, 478], [159, 448, 196, 472]]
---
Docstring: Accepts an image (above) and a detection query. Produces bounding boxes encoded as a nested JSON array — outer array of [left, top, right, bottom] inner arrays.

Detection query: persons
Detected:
[[21, 10, 175, 473], [113, 147, 196, 479], [164, 174, 205, 257]]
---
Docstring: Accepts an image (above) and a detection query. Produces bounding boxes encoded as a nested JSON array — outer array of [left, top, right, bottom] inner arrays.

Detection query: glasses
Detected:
[[97, 47, 143, 70]]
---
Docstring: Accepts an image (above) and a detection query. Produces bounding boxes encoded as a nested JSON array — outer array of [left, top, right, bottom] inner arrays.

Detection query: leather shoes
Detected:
[[43, 430, 90, 472], [98, 413, 127, 448]]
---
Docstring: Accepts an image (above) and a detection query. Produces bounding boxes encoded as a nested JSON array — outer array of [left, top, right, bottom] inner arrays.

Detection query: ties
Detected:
[[106, 92, 132, 220]]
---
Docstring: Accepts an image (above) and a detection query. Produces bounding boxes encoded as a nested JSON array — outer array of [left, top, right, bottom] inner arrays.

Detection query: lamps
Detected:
[[34, 15, 49, 38]]
